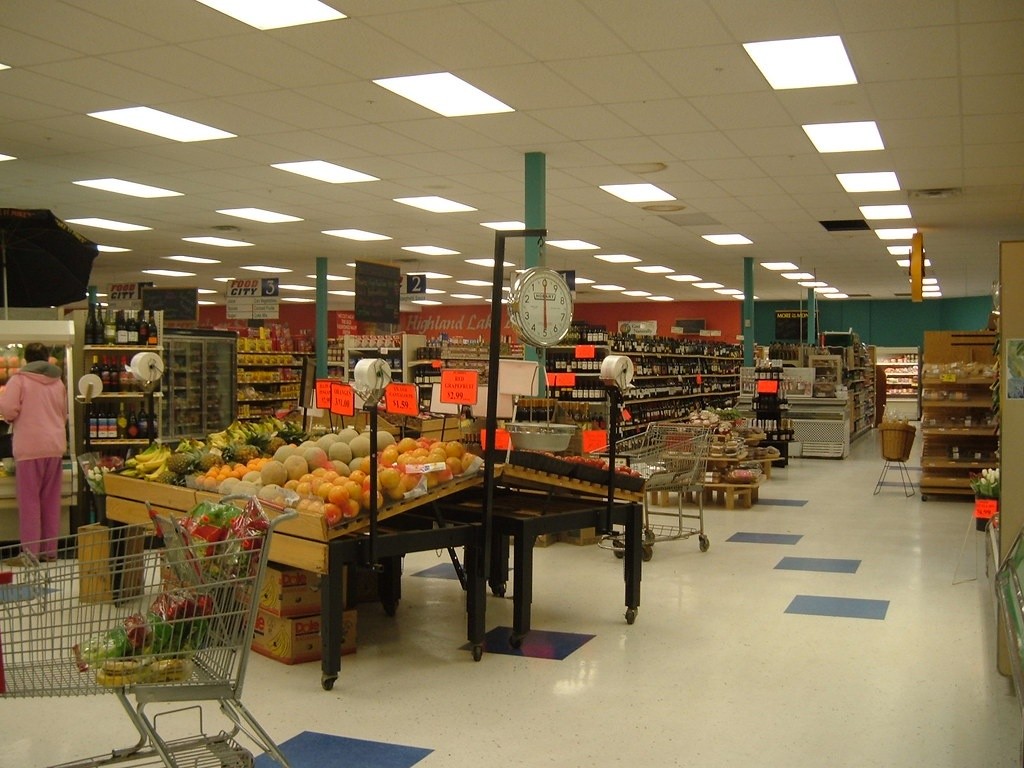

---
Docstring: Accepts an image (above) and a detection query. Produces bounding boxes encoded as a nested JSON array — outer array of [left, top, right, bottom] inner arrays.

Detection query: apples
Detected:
[[519, 449, 640, 477], [49, 357, 57, 365], [0, 356, 26, 381]]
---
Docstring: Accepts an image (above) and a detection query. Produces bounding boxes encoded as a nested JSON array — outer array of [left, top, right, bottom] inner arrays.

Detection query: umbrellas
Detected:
[[0, 208, 99, 308]]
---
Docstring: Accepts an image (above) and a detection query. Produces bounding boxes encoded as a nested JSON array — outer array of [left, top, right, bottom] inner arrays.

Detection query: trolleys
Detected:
[[586, 418, 722, 562], [0, 492, 292, 768]]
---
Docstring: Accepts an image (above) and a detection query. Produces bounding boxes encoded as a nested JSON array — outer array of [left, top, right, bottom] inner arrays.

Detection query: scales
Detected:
[[504, 262, 577, 451]]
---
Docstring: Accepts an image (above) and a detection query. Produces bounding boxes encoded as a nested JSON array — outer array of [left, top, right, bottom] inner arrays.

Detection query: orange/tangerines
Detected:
[[285, 437, 476, 524], [197, 457, 273, 487]]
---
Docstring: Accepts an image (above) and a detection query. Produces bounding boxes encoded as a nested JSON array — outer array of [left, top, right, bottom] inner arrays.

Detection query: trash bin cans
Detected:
[[77, 522, 145, 604]]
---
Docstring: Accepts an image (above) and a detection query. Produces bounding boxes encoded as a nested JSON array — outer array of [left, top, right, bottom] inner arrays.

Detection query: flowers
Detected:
[[969, 467, 1001, 499]]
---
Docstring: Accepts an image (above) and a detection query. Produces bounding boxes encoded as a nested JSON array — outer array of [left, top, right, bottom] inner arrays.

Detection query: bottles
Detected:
[[413, 346, 442, 413], [76, 285, 159, 524], [742, 340, 833, 410], [748, 418, 796, 440], [517, 325, 744, 455]]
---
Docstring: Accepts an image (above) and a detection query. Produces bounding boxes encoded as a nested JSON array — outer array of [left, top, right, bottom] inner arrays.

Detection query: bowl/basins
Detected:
[[505, 423, 578, 451]]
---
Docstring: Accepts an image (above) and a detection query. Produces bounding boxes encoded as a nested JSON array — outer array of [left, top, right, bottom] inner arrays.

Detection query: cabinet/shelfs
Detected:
[[77, 309, 164, 446], [760, 343, 882, 441], [874, 347, 918, 420], [920, 359, 999, 503], [540, 342, 748, 453], [238, 332, 523, 426]]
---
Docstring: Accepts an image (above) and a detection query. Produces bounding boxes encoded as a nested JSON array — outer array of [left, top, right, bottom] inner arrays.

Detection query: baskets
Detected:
[[878, 423, 916, 462]]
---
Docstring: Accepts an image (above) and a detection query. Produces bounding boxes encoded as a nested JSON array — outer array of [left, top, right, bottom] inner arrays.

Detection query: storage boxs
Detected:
[[508, 525, 605, 550], [248, 603, 362, 662], [232, 562, 356, 616], [69, 523, 145, 602]]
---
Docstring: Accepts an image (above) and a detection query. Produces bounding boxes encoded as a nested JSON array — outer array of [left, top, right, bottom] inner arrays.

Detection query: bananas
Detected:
[[95, 658, 190, 686], [120, 416, 282, 480]]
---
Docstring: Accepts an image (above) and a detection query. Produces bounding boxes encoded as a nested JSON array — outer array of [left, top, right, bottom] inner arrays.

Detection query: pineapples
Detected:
[[154, 436, 287, 484]]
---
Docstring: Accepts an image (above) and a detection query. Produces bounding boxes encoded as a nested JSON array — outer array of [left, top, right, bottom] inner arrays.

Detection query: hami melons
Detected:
[[220, 428, 395, 501]]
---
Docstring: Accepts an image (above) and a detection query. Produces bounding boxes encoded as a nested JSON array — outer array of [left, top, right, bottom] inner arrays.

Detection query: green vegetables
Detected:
[[79, 532, 248, 667], [708, 406, 741, 420]]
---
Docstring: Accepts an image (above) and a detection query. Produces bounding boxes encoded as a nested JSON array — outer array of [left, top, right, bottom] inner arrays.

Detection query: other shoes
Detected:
[[7, 554, 39, 567], [40, 555, 57, 562]]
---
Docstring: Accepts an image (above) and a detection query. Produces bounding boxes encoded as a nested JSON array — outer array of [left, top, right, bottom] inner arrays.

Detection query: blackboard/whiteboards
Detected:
[[673, 318, 707, 335], [355, 259, 400, 324], [775, 309, 819, 340]]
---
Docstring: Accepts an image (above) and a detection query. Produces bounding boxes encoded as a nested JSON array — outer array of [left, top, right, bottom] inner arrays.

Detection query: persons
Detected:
[[0, 342, 68, 567]]
